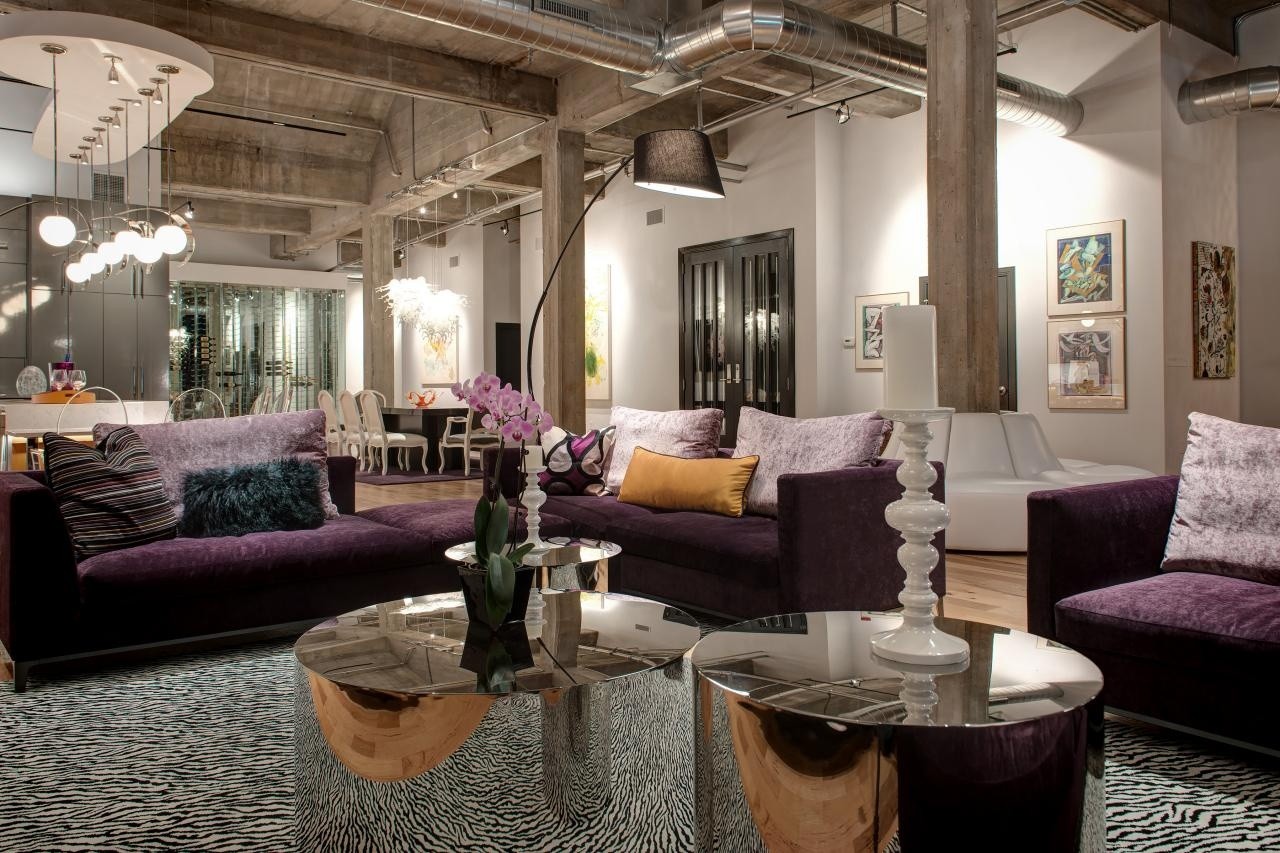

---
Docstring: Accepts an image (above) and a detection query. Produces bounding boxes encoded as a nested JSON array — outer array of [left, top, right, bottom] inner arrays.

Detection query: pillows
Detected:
[[731, 406, 894, 517], [617, 445, 760, 518], [538, 425, 616, 497], [596, 406, 724, 497], [92, 410, 339, 536], [1159, 411, 1280, 586], [43, 426, 178, 562]]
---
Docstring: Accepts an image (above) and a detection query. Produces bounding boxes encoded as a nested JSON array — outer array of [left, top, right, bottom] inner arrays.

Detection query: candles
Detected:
[[882, 304, 940, 408], [519, 446, 542, 466]]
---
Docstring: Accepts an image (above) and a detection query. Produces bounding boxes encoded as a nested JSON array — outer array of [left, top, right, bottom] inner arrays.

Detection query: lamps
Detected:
[[66, 64, 187, 284], [501, 221, 509, 236], [39, 43, 76, 247], [835, 100, 850, 125], [374, 185, 469, 348], [171, 201, 194, 220], [526, 129, 727, 401], [104, 55, 123, 85]]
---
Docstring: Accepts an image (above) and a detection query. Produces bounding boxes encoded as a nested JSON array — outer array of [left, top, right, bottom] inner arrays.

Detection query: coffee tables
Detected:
[[688, 610, 1105, 853], [292, 588, 703, 853]]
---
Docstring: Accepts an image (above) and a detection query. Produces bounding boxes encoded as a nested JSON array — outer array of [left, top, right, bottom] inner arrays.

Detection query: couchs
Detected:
[[1028, 475, 1280, 757], [482, 445, 947, 621], [0, 456, 573, 693]]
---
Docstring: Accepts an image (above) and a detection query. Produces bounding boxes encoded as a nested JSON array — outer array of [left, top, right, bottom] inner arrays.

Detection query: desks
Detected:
[[0, 399, 169, 435]]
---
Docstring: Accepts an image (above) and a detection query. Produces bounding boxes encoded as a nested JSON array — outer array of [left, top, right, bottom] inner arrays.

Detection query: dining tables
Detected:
[[381, 407, 469, 472]]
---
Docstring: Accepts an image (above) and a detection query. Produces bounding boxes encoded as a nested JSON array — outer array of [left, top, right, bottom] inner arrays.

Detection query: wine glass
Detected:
[[51, 369, 86, 391]]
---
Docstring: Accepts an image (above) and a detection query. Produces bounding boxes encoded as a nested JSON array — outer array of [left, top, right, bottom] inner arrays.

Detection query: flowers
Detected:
[[451, 371, 555, 633]]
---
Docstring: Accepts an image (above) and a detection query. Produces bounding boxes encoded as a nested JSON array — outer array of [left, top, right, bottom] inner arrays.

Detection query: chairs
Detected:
[[360, 392, 428, 476], [438, 406, 504, 476], [340, 390, 404, 472], [318, 390, 359, 460], [353, 390, 386, 456]]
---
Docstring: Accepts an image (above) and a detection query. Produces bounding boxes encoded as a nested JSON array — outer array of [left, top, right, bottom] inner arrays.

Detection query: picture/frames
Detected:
[[1047, 317, 1127, 409], [1046, 219, 1126, 316], [855, 292, 910, 368]]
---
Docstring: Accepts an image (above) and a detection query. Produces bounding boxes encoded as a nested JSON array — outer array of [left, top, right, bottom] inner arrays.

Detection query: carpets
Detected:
[[0, 637, 1280, 853]]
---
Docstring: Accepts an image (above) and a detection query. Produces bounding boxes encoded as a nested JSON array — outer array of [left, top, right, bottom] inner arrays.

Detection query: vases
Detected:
[[456, 565, 535, 674]]
